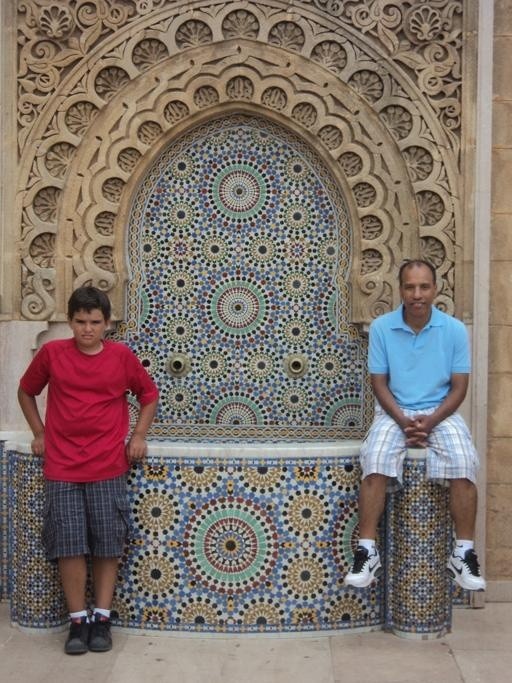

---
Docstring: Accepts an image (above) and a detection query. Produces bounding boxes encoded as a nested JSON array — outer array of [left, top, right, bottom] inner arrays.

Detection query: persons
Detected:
[[17, 286, 159, 656], [343, 258, 487, 593]]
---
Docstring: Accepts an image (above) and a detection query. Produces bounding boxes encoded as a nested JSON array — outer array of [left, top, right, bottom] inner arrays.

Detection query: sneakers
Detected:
[[447, 549, 486, 591], [344, 546, 384, 587], [65, 613, 111, 654]]
